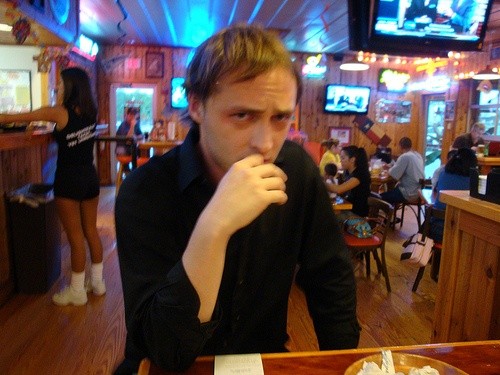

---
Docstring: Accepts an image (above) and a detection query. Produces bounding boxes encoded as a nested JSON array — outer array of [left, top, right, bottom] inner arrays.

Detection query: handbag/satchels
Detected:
[[400, 205, 435, 267], [343, 218, 374, 239]]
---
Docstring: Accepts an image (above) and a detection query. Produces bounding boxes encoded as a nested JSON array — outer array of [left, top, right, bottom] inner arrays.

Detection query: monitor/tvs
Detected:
[[345, 0, 493, 60], [323, 84, 371, 115], [169, 76, 187, 109]]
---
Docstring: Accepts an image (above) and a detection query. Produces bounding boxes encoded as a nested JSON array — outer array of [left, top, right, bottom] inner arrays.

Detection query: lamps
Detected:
[[340, 56, 369, 70], [473, 27, 500, 79]]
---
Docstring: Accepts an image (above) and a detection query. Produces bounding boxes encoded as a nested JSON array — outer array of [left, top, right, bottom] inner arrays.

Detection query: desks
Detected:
[[142, 142, 175, 155]]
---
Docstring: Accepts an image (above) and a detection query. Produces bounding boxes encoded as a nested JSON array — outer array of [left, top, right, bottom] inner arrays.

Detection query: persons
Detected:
[[324, 162, 338, 199], [397, 0, 477, 36], [426, 148, 482, 244], [0, 68, 106, 305], [324, 145, 371, 273], [449, 122, 486, 153], [112, 24, 362, 374], [368, 136, 423, 228], [115, 107, 143, 176], [337, 90, 364, 108], [318, 137, 344, 180]]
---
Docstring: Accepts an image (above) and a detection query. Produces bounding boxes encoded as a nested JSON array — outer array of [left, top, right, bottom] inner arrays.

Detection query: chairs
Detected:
[[340, 179, 446, 293]]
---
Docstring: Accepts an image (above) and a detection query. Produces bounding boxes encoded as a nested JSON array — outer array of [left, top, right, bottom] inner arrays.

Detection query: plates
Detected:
[[344, 353, 468, 375]]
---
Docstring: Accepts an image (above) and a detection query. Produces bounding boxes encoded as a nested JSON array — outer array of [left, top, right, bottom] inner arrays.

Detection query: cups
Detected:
[[478, 145, 485, 155]]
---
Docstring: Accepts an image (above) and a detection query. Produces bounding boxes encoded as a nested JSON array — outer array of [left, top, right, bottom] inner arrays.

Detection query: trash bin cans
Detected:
[[5, 182, 62, 296]]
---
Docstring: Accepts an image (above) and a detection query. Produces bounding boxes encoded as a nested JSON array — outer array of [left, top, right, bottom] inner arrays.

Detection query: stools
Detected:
[[118, 155, 147, 192]]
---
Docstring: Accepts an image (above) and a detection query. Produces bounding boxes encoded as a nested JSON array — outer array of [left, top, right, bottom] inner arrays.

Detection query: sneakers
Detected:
[[84, 278, 106, 296], [52, 285, 87, 306]]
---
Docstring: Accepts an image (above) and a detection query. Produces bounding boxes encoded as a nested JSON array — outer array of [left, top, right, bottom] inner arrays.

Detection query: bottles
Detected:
[[484, 142, 489, 157]]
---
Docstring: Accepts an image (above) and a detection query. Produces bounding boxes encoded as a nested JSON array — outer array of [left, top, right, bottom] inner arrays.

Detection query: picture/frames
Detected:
[[329, 127, 352, 145], [145, 52, 164, 78]]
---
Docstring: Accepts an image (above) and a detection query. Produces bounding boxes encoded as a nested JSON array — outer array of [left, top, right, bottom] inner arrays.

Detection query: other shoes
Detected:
[[383, 217, 400, 224]]
